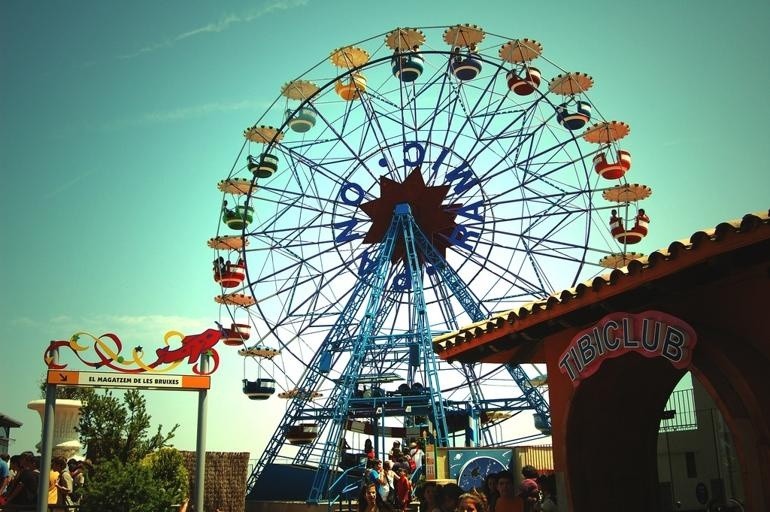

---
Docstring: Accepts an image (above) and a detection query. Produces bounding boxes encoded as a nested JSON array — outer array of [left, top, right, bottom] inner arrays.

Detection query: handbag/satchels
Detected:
[[408, 459, 416, 474], [388, 487, 398, 506]]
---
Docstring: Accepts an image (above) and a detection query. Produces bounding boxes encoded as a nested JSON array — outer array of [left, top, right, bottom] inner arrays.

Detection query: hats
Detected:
[[521, 479, 538, 491], [410, 443, 416, 447]]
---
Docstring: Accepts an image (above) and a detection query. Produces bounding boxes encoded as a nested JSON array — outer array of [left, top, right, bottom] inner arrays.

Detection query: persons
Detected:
[[636, 209, 649, 219], [413, 45, 423, 58], [453, 46, 462, 63], [216, 256, 231, 273], [0, 449, 95, 512], [358, 426, 435, 512], [611, 209, 618, 221], [435, 465, 558, 511], [392, 47, 400, 59], [469, 44, 480, 54]]
